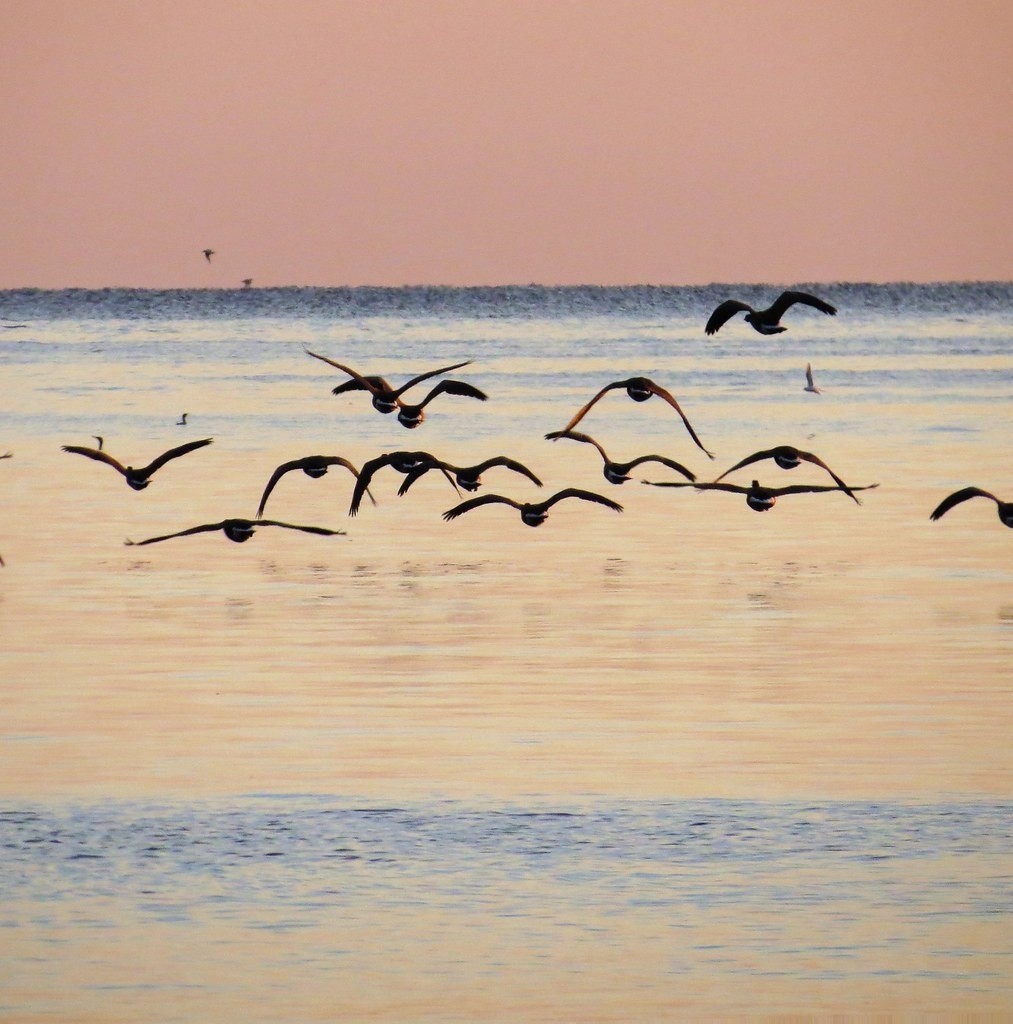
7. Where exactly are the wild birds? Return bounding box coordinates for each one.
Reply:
[397,455,543,498]
[442,488,625,527]
[543,431,697,485]
[803,363,825,395]
[304,346,474,414]
[714,445,862,506]
[331,376,488,429]
[256,456,378,520]
[57,438,215,491]
[347,451,463,518]
[929,485,1013,530]
[641,478,880,512]
[138,518,340,546]
[565,377,717,461]
[703,291,838,337]
[176,414,188,425]
[202,249,214,263]
